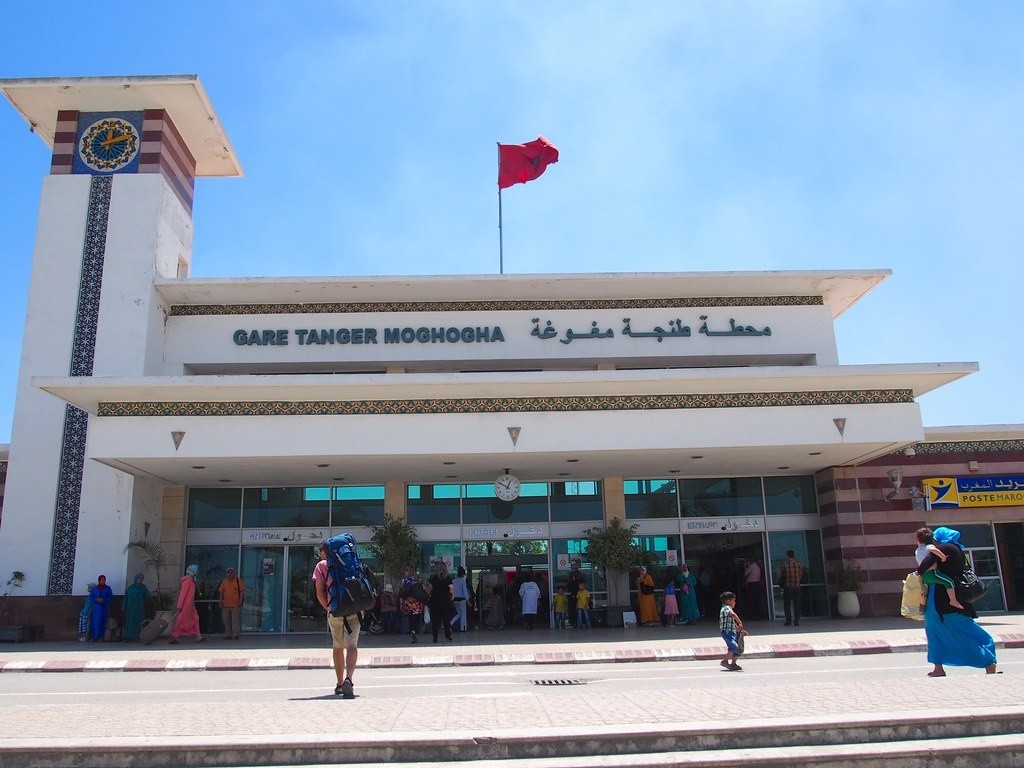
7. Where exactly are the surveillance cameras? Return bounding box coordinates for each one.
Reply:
[905,447,916,459]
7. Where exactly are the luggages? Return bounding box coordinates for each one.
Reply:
[139,609,182,644]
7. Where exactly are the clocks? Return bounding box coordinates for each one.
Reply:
[78,116,140,171]
[494,474,521,501]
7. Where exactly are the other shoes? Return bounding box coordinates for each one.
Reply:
[720,660,733,670]
[784,621,791,626]
[732,663,742,670]
[794,620,798,625]
[410,632,418,643]
[79,636,88,642]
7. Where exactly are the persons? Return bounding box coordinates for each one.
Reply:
[78,583,96,641]
[89,575,112,642]
[449,566,473,634]
[780,549,803,625]
[427,562,454,643]
[637,566,658,627]
[677,564,701,626]
[313,546,365,700]
[717,592,749,671]
[576,582,592,628]
[915,528,998,678]
[662,578,678,629]
[218,567,247,640]
[689,559,763,622]
[567,559,586,625]
[552,586,569,630]
[379,583,398,636]
[400,562,423,643]
[121,573,154,643]
[167,564,208,644]
[484,571,546,628]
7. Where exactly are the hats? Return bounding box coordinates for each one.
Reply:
[87,582,96,589]
[227,567,235,573]
[383,584,393,593]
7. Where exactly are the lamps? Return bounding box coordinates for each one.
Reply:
[884,467,903,502]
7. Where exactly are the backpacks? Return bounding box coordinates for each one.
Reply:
[323,533,377,617]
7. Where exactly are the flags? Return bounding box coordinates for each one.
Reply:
[498,135,559,189]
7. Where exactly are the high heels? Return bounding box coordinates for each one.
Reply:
[433,636,437,642]
[446,636,453,641]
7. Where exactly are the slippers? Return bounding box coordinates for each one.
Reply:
[196,637,207,643]
[167,638,179,645]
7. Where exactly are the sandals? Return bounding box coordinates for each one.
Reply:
[342,677,355,699]
[335,685,343,693]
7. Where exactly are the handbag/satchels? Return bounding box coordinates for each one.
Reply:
[368,621,384,634]
[424,605,430,625]
[400,582,412,599]
[901,573,928,620]
[640,576,653,594]
[412,584,428,601]
[953,570,988,604]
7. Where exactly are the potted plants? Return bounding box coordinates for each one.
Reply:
[0,571,25,643]
[580,514,660,628]
[832,564,865,618]
[119,538,174,637]
[370,516,423,632]
